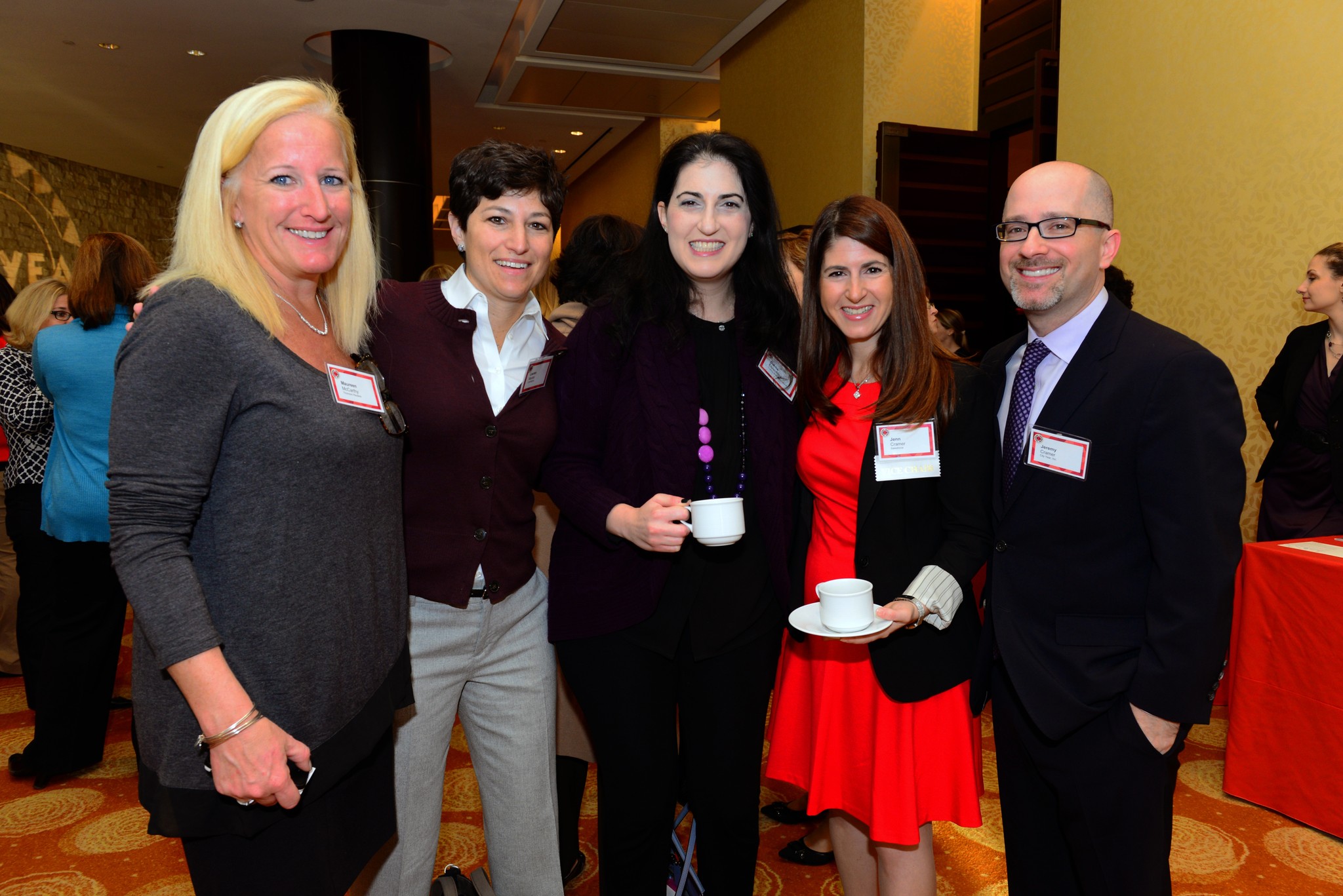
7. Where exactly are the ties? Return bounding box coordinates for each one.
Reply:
[994,339,1054,518]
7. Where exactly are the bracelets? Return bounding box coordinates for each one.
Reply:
[894,594,925,632]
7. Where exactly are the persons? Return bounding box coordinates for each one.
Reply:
[921,289,938,341]
[97,82,416,896]
[759,199,990,896]
[1249,241,1343,542]
[2,221,840,883]
[937,309,979,380]
[355,138,572,896]
[971,158,1247,895]
[544,123,809,896]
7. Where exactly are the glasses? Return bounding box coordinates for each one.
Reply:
[994,217,1114,244]
[47,308,75,322]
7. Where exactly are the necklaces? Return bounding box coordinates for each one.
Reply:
[1325,329,1343,360]
[844,360,881,401]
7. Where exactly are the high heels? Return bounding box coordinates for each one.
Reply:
[777,829,835,869]
[760,788,828,826]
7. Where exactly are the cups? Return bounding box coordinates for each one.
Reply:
[680,497,745,547]
[815,579,874,633]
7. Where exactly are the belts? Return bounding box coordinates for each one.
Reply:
[468,585,490,602]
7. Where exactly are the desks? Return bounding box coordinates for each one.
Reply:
[1219,536,1342,841]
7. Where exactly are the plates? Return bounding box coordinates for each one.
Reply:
[788,602,894,637]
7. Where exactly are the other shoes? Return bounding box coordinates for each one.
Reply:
[7,738,36,779]
[28,744,109,791]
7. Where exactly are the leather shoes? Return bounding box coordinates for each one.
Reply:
[555,848,586,889]
